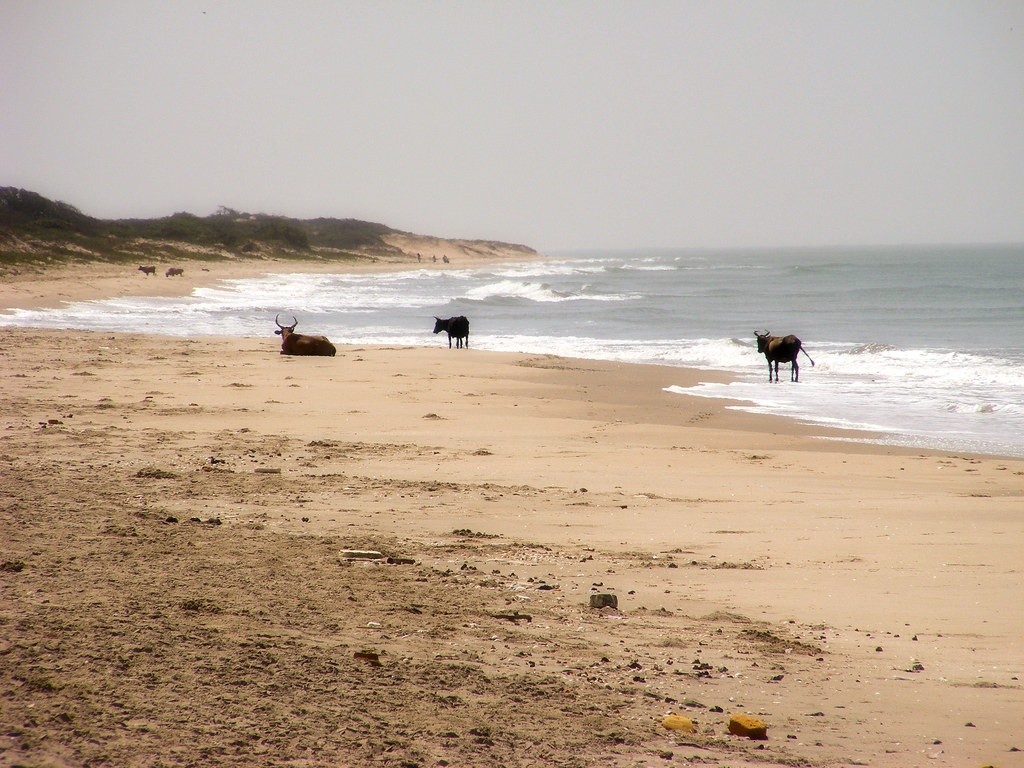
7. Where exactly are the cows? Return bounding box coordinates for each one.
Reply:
[754,329,815,382]
[274,313,337,357]
[433,316,469,349]
[138,265,184,277]
